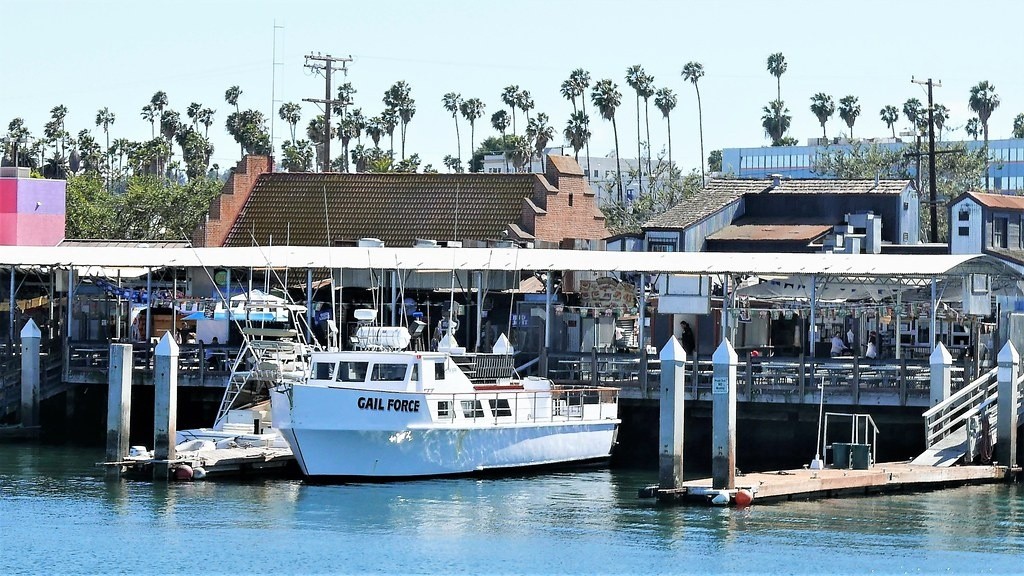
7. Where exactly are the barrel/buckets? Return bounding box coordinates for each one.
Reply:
[850,443,871,470]
[832,444,849,469]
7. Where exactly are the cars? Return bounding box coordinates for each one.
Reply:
[139,307,197,339]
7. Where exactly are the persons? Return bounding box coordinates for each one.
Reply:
[205,337,222,371]
[677,321,695,355]
[865,331,883,359]
[176,322,195,344]
[831,331,848,357]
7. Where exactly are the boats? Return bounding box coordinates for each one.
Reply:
[127,230,356,479]
[269,275,622,485]
